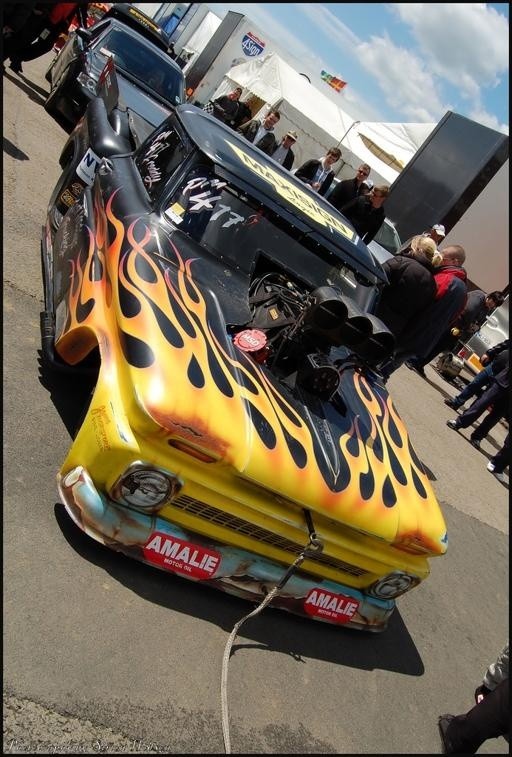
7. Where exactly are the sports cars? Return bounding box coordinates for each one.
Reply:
[38,96,454,637]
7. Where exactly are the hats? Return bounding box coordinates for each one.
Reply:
[432,223,446,239]
[285,131,298,143]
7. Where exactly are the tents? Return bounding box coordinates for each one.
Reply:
[203,50,403,212]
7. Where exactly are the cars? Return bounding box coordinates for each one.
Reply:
[295,163,402,266]
[43,2,187,131]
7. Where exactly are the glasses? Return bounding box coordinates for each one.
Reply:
[358,169,368,177]
[371,190,384,198]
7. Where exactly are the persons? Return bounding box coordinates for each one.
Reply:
[475,642,509,706]
[1,0,88,78]
[437,675,512,756]
[214,88,509,477]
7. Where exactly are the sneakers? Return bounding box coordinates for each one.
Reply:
[493,470,506,484]
[446,419,460,431]
[443,398,459,412]
[414,366,427,378]
[439,713,455,753]
[487,459,496,473]
[471,437,481,449]
[404,359,414,370]
